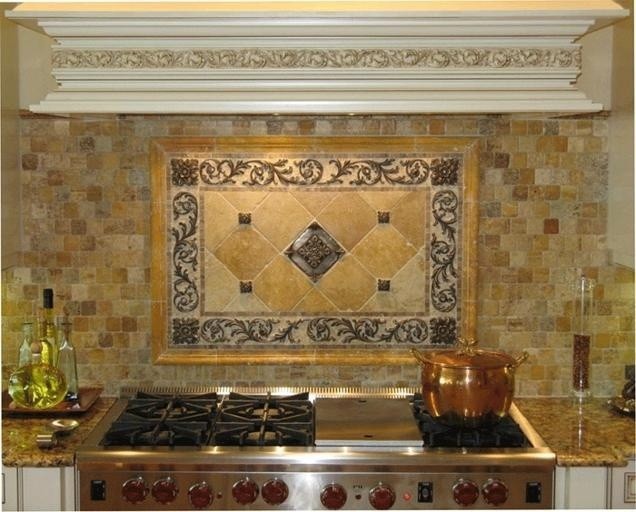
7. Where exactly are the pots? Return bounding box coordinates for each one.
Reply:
[405,336,530,420]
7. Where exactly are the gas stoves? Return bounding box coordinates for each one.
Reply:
[76,386,557,511]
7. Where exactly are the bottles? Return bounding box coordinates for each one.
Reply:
[567,275,596,404]
[7,286,78,410]
